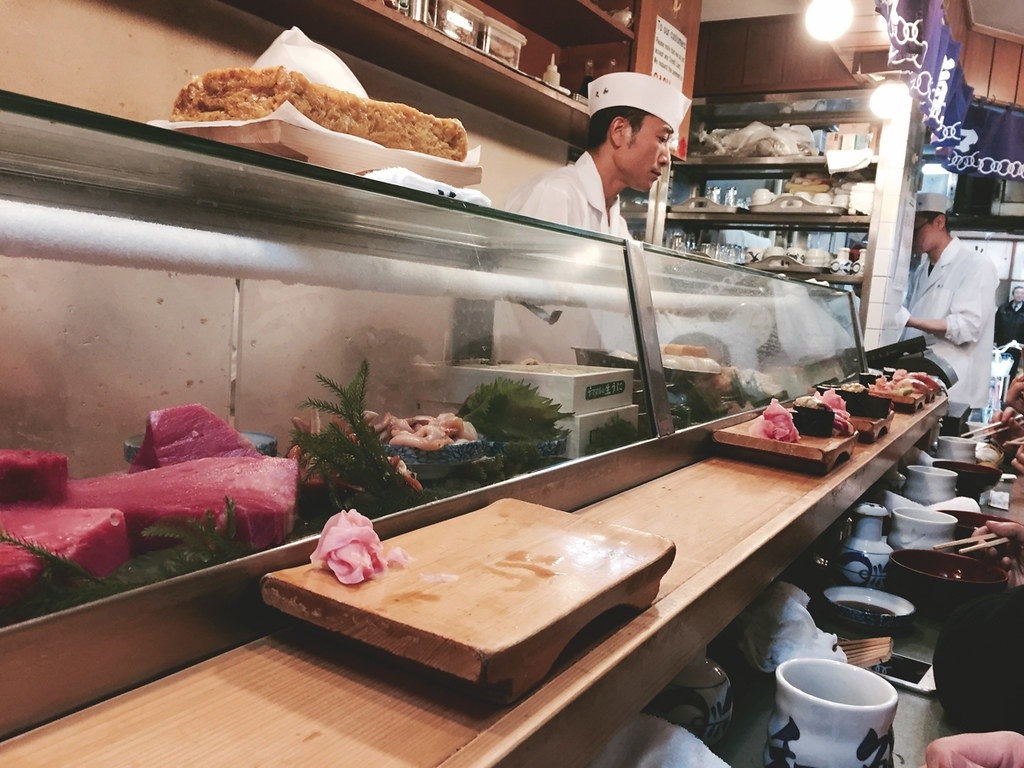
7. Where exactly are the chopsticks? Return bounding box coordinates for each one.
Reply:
[960,414,1024,441]
[933,533,1012,555]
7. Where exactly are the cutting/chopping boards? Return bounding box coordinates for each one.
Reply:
[261,497,678,701]
[171,123,484,187]
[711,369,945,476]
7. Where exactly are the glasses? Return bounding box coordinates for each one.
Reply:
[914,219,930,237]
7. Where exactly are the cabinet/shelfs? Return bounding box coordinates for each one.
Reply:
[652,102,885,341]
[218,0,697,156]
[1,87,952,767]
[620,180,657,243]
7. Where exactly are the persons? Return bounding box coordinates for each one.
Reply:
[491,72,695,366]
[994,286,1024,346]
[925,371,1024,768]
[901,171,998,410]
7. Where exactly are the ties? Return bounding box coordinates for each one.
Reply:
[1013,304,1017,314]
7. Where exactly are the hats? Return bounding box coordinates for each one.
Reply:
[912,163,958,214]
[587,72,692,136]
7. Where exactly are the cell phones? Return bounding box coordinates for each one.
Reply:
[871,651,937,695]
[1000,473,1018,484]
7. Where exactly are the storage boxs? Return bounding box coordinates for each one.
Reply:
[436,0,482,50]
[478,17,527,69]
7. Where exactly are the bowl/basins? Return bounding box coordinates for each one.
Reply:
[901,464,959,509]
[823,583,918,634]
[888,547,1007,622]
[932,460,1003,503]
[762,661,896,768]
[886,508,960,555]
[935,435,978,465]
